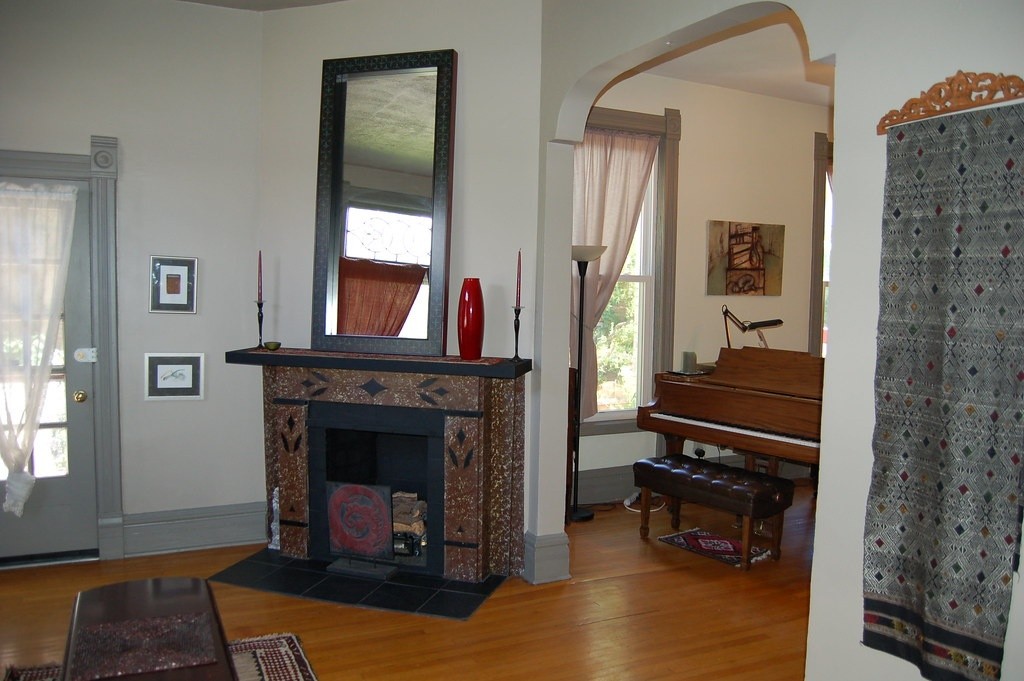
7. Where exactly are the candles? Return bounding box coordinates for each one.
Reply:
[255,248,266,302]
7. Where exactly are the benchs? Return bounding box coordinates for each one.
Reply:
[631,449,795,567]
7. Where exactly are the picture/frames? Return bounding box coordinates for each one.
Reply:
[142,352,206,401]
[704,220,787,298]
[146,252,200,316]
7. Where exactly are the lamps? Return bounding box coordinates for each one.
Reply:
[568,243,609,526]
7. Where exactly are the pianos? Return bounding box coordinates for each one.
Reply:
[637,345,826,513]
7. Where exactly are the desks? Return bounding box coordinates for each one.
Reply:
[61,575,244,681]
[223,339,532,588]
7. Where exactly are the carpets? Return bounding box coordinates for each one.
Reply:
[2,632,320,681]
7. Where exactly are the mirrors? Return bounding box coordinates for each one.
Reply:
[310,47,460,355]
